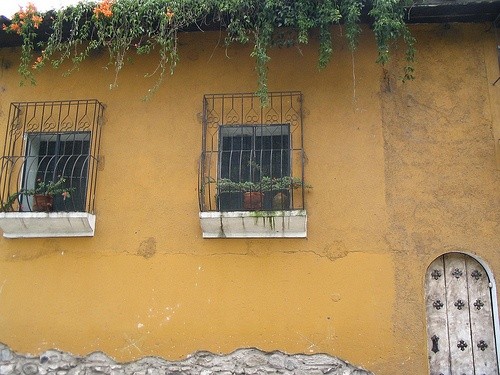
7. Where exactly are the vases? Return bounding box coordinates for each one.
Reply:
[31,195,53,212]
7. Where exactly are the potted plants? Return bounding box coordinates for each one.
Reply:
[263,175,313,209]
[199,174,269,210]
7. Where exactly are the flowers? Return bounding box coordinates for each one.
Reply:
[2,175,78,209]
